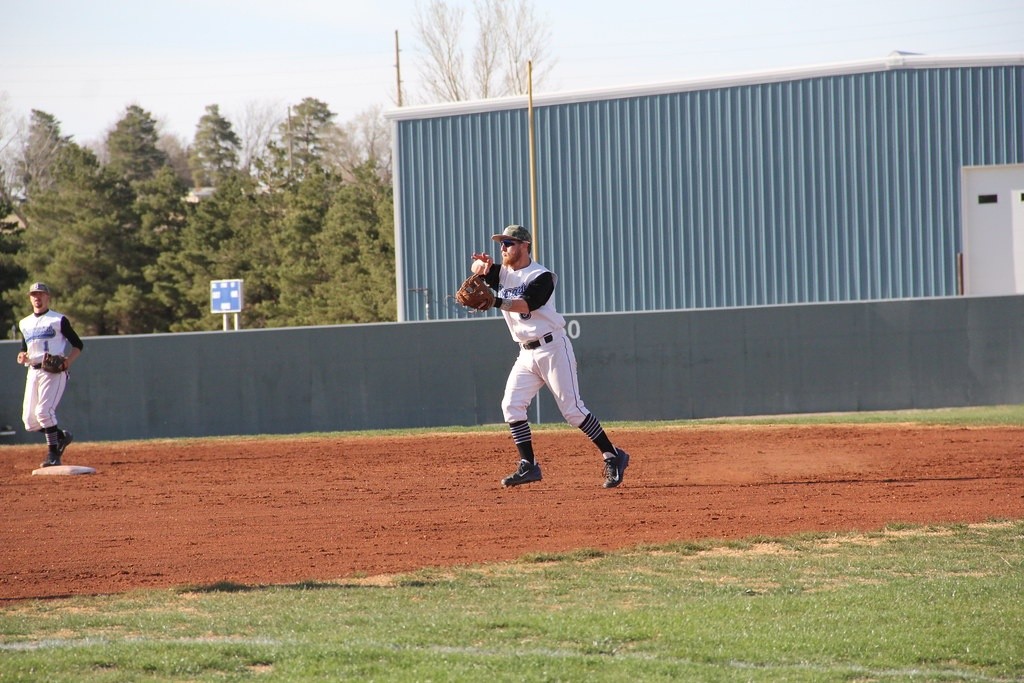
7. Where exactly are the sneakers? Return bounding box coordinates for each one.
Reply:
[501,459,541,488]
[602,448,629,488]
[57,430,72,456]
[41,451,60,467]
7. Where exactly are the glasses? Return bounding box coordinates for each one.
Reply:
[500,239,530,247]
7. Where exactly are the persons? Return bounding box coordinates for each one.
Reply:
[455,224,629,488]
[17,282,84,468]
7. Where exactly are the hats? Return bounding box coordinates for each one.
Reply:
[492,225,532,244]
[29,283,48,292]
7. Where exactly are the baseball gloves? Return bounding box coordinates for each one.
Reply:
[454,273,495,311]
[41,352,68,374]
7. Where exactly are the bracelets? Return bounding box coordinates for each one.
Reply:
[500,298,512,311]
[493,297,502,308]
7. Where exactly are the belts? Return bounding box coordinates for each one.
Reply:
[33,364,41,369]
[523,335,552,349]
